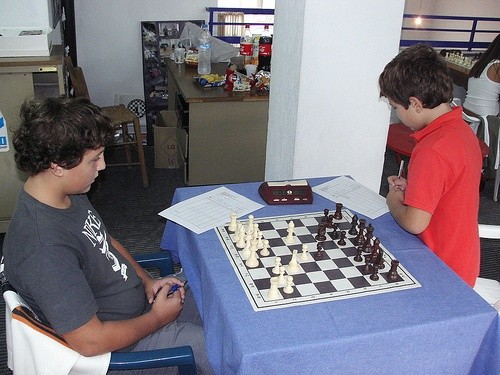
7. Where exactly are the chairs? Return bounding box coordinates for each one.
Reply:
[65,56,149,190]
[449,97,500,202]
[472,224,500,314]
[0,249,198,375]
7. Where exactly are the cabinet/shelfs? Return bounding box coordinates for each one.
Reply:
[0,41,69,232]
[164,56,269,187]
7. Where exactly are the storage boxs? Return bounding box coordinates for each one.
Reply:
[51,16,64,47]
[0,27,53,58]
[152,109,179,170]
[0,0,62,30]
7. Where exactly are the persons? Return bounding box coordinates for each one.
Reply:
[462,34,500,183]
[378,42,483,289]
[3,94,213,375]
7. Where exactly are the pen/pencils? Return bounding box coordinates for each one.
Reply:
[397,159,404,178]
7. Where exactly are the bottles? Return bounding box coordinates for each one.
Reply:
[198,25,211,75]
[258,25,272,72]
[240,24,253,64]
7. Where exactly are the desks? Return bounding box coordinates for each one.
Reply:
[387,123,490,177]
[158,175,500,375]
[438,50,485,88]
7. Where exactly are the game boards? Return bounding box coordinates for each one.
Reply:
[213,209,422,312]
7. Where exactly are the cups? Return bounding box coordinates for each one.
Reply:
[231,56,243,70]
[174,48,185,63]
[245,65,257,76]
